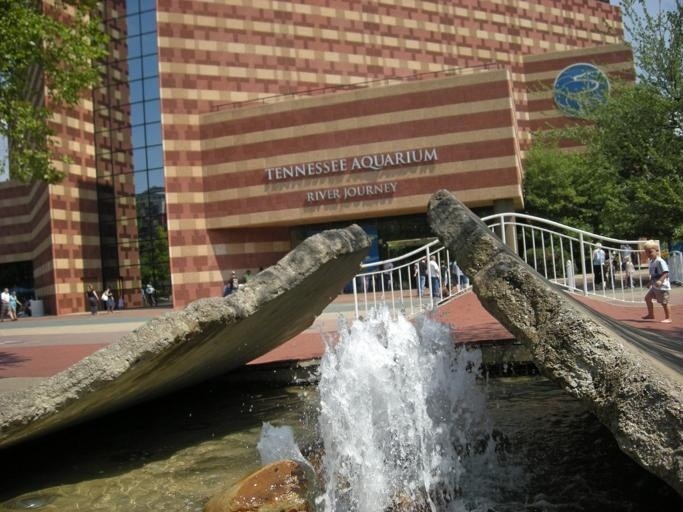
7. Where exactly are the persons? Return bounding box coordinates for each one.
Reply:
[146,280,158,307]
[383,263,393,290]
[86,283,126,315]
[223,266,266,296]
[619,243,635,289]
[0,288,14,321]
[566,256,575,292]
[639,245,673,323]
[10,291,22,320]
[592,242,607,291]
[413,253,470,298]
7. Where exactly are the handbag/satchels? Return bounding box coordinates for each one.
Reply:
[101,293,108,301]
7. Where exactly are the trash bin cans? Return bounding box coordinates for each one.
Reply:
[27,299,43,317]
